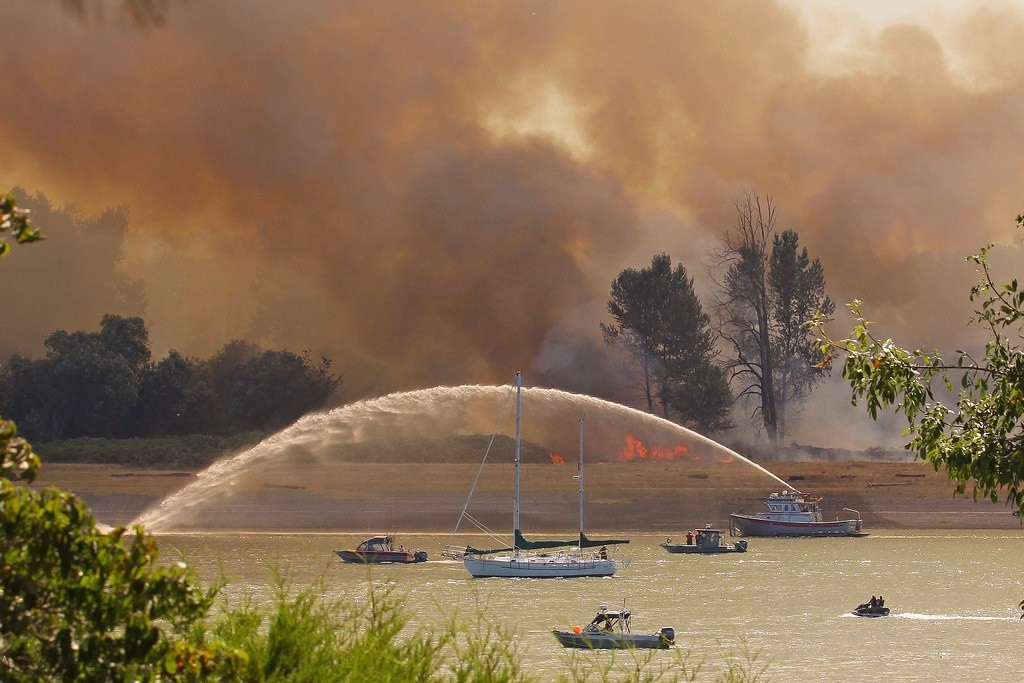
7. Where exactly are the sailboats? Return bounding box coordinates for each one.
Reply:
[440,367,631,580]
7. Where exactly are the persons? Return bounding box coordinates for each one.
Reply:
[399,545,405,553]
[598,545,610,560]
[685,531,703,545]
[868,595,885,610]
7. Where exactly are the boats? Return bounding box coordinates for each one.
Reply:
[332,534,430,565]
[726,488,871,538]
[852,603,892,619]
[657,522,749,556]
[550,604,677,651]
